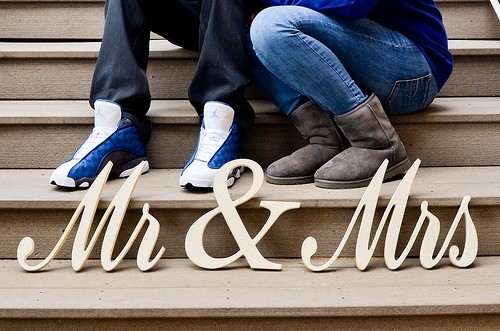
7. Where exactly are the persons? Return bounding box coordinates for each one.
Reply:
[51,1,268,189]
[245,0,453,190]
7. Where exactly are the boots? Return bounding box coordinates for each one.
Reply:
[265,92,412,189]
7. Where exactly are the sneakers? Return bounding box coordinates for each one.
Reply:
[49,98,149,188]
[180,101,244,191]
